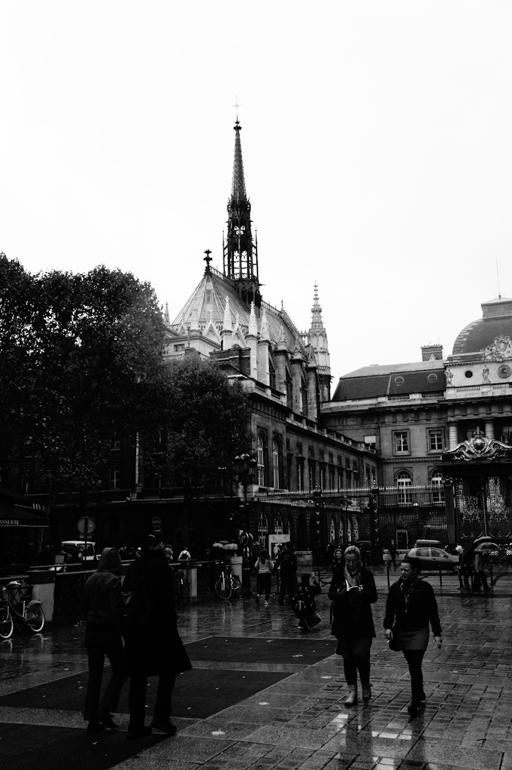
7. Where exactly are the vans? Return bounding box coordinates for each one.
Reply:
[61,540,96,560]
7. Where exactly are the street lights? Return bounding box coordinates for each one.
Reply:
[234,453,258,598]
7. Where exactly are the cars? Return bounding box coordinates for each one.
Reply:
[405,547,461,574]
[474,536,512,561]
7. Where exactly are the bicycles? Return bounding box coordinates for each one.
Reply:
[0,581,44,638]
[215,565,233,600]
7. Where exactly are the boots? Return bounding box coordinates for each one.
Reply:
[152,700,179,734]
[361,678,372,700]
[344,685,357,704]
[128,709,153,739]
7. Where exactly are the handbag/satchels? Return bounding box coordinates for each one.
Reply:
[388,622,403,652]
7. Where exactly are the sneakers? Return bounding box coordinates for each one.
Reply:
[100,713,121,729]
[87,721,105,733]
[407,697,426,716]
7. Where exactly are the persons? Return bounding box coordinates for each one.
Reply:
[272,546,296,606]
[285,541,298,571]
[122,532,196,740]
[386,543,399,569]
[328,545,379,706]
[254,550,276,606]
[382,556,442,714]
[177,543,193,579]
[288,572,322,632]
[81,546,127,733]
[473,550,488,583]
[331,548,345,579]
[457,543,474,591]
